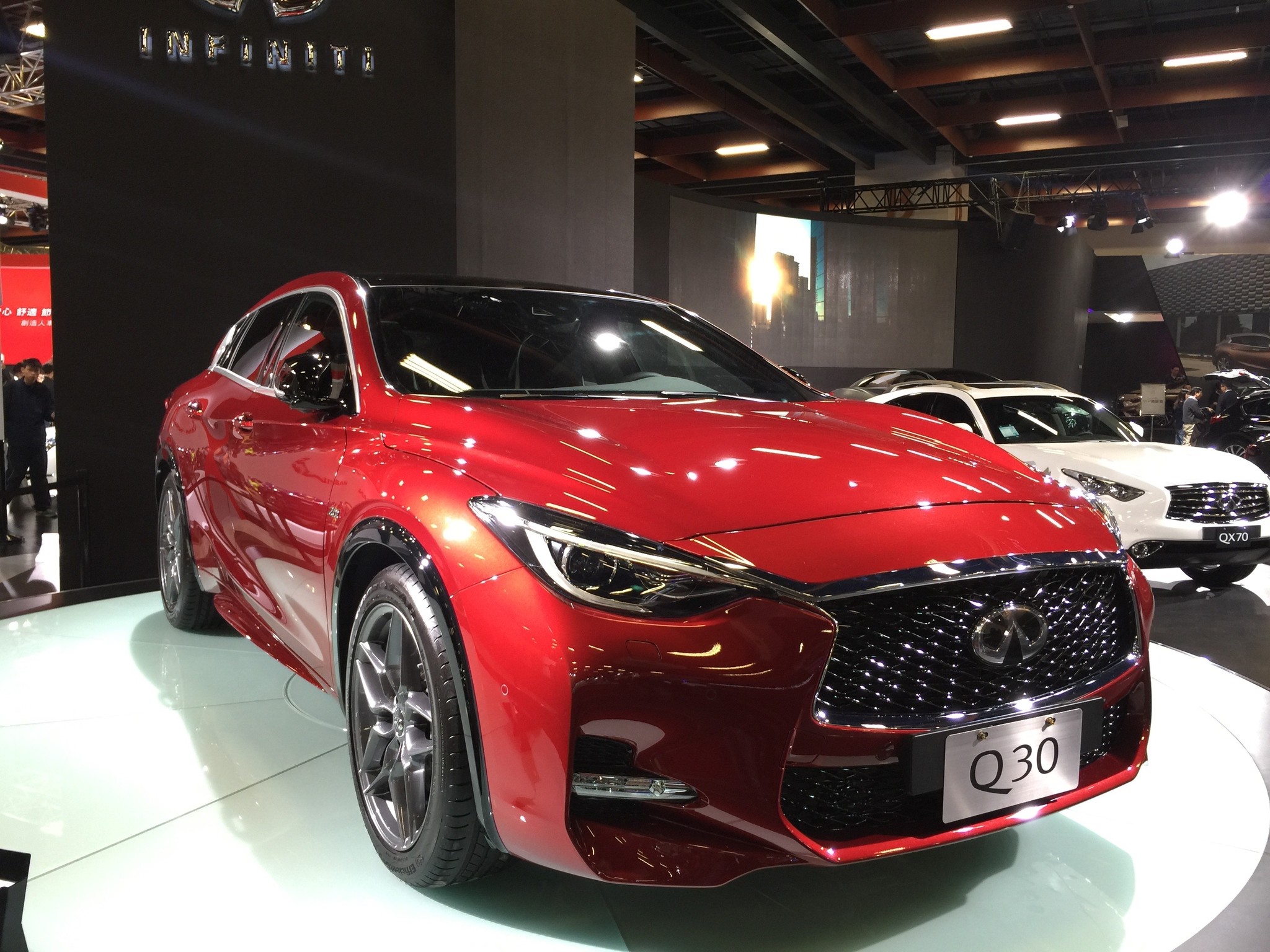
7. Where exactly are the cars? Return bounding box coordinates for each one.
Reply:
[1201,368,1270,400]
[829,367,1004,403]
[1110,377,1192,429]
[155,269,1158,898]
[25,423,57,498]
[1212,332,1270,379]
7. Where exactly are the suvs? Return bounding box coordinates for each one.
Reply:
[1203,389,1270,477]
[862,378,1270,588]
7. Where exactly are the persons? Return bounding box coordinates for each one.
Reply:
[1173,389,1191,446]
[1181,386,1216,446]
[4,362,26,387]
[1164,364,1185,390]
[40,363,56,428]
[2,357,59,518]
[1182,384,1193,391]
[1213,379,1238,415]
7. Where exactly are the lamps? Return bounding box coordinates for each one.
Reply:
[1056,197,1077,233]
[1086,193,1109,231]
[1124,192,1155,234]
[1162,49,1249,68]
[715,142,770,158]
[634,70,644,84]
[995,111,1062,127]
[924,14,1014,42]
